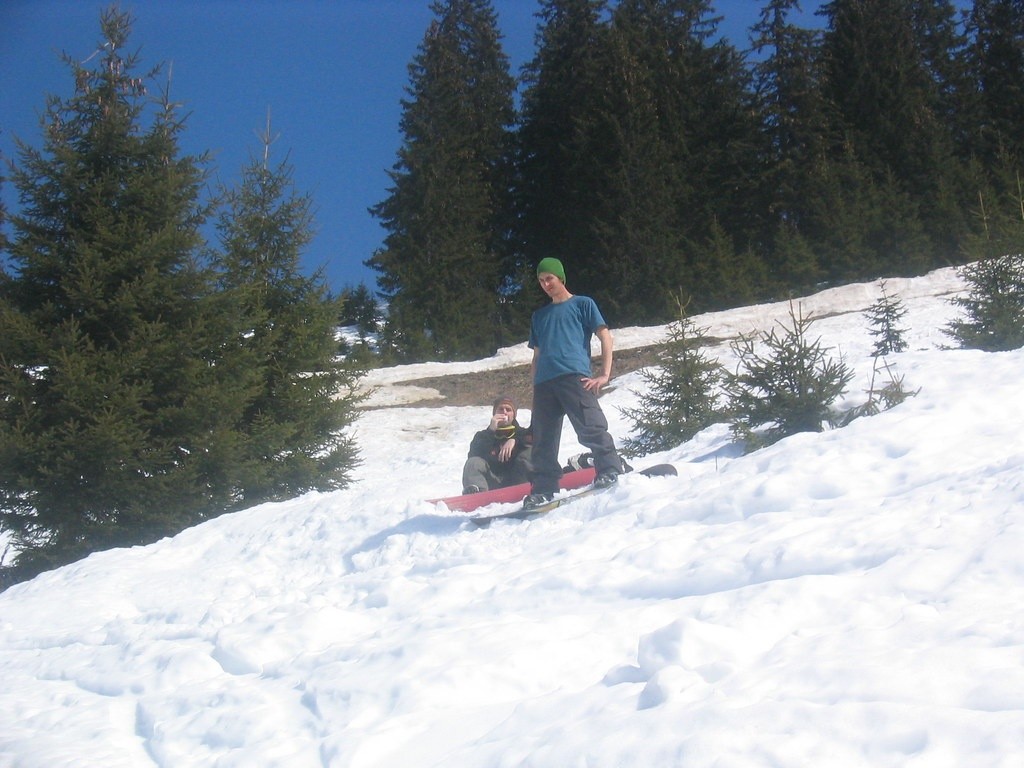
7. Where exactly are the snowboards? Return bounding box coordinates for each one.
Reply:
[468,477,621,527]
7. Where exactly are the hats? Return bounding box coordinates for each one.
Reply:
[493,396,517,418]
[537,257,566,284]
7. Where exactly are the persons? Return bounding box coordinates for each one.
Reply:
[463,396,535,494]
[523,257,614,511]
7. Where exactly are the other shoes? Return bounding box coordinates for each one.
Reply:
[462,485,479,495]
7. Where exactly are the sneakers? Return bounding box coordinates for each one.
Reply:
[523,493,552,508]
[594,472,619,489]
